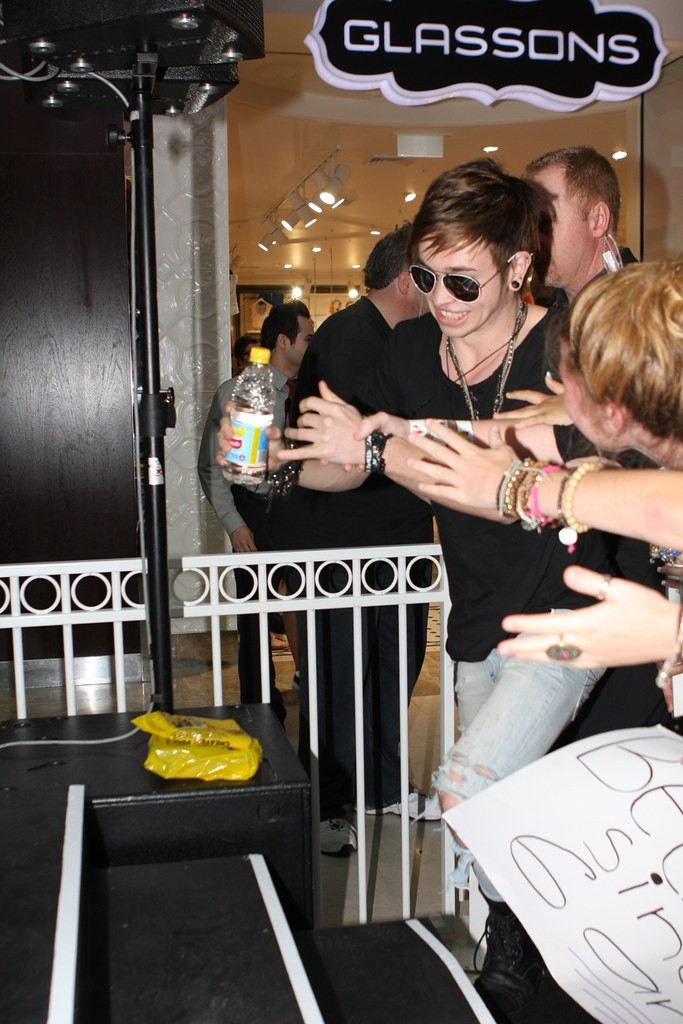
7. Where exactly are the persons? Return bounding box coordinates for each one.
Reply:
[284,225,444,859]
[214,157,648,1024]
[235,335,259,377]
[198,300,317,699]
[524,145,646,744]
[321,258,683,1023]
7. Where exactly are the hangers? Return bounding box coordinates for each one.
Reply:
[249,294,274,308]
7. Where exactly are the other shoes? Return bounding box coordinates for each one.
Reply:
[293,671,301,690]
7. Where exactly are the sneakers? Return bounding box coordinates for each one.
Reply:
[270,632,289,649]
[355,782,442,819]
[320,816,358,855]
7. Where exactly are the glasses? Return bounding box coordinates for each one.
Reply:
[408,251,522,303]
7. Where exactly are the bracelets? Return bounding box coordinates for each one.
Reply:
[274,436,303,498]
[498,459,561,527]
[409,418,427,443]
[456,420,473,444]
[555,461,607,537]
[432,417,448,445]
[363,429,389,475]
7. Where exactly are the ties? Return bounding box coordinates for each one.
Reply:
[285,378,297,427]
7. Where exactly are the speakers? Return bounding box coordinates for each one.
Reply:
[0,0,265,115]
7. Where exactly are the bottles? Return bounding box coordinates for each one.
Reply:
[221,346,276,483]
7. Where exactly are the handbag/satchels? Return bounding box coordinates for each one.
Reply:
[129,712,263,780]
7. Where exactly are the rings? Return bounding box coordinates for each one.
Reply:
[217,450,225,457]
[597,574,611,600]
[544,632,582,660]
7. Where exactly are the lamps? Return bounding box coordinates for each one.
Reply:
[257,145,347,251]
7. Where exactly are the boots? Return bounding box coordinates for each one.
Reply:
[474,885,544,1014]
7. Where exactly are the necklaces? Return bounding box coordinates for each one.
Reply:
[442,298,528,427]
